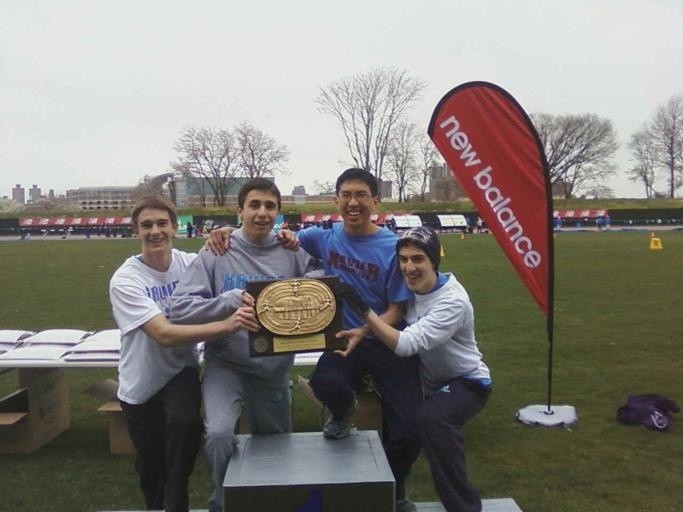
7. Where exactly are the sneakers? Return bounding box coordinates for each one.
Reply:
[323,391,357,440]
[396,498,416,512]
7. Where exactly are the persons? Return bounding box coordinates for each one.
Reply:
[595,214,611,234]
[17,216,493,235]
[556,215,563,229]
[108,194,300,512]
[203,166,424,511]
[166,176,323,512]
[331,226,493,512]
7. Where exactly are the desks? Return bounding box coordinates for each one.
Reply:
[1,351,322,366]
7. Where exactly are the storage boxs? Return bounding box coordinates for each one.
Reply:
[81,376,139,455]
[0,367,70,455]
[296,373,384,432]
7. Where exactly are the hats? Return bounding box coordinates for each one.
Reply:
[398,227,442,265]
